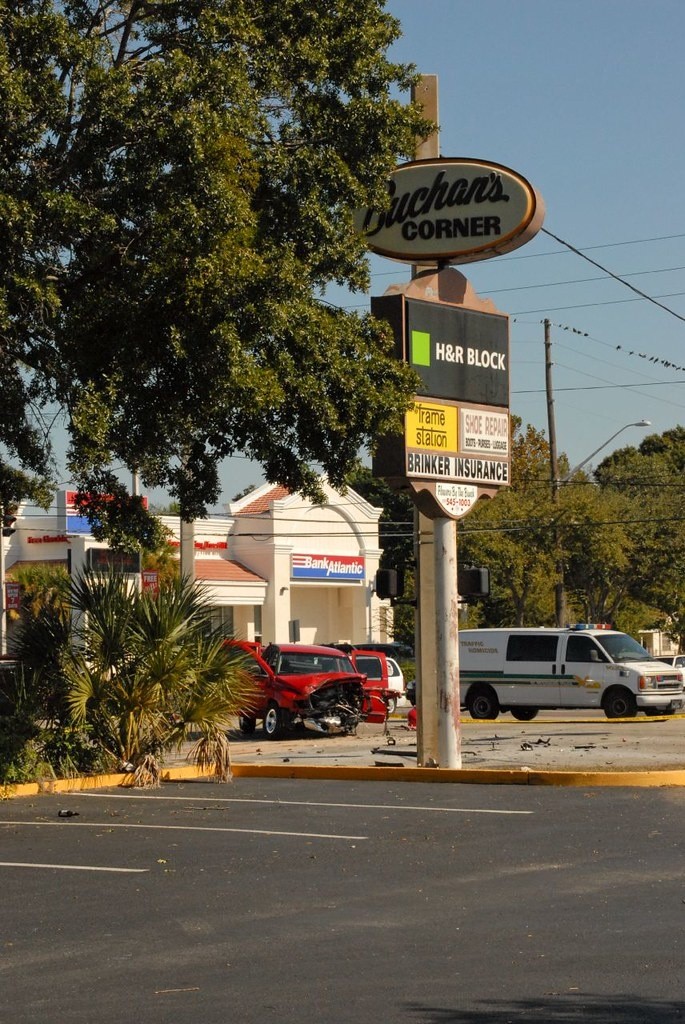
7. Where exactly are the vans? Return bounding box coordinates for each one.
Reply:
[458,624,685,722]
[314,654,404,715]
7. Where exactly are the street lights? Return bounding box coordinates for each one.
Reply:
[546,420,651,628]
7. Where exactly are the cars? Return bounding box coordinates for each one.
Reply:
[653,655,685,688]
[218,639,402,739]
[405,680,415,707]
